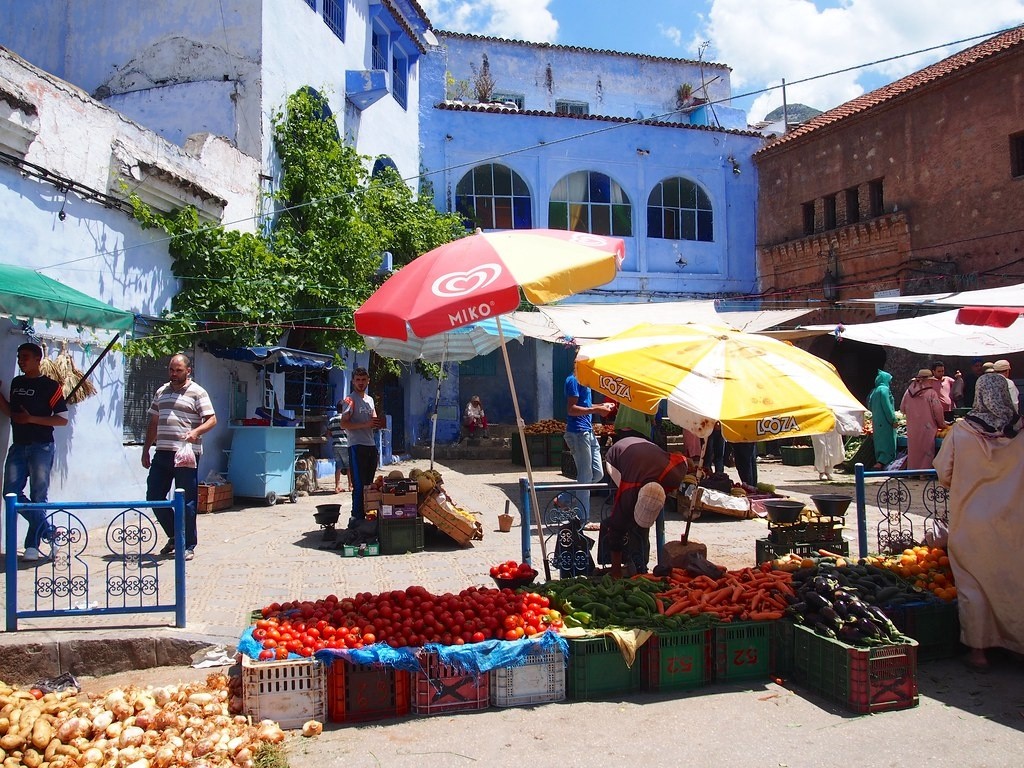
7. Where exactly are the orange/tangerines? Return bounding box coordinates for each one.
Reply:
[890,544,959,600]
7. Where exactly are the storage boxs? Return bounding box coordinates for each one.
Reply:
[512,432,875,478]
[241,478,965,731]
[953,407,973,421]
[197,483,232,514]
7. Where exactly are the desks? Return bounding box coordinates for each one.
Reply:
[896,435,944,480]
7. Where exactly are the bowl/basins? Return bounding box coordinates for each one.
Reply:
[489,570,539,591]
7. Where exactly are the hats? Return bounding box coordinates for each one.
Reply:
[982,362,994,373]
[911,368,938,382]
[634,483,665,529]
[994,360,1012,371]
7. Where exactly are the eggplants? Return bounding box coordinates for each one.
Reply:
[769,565,906,647]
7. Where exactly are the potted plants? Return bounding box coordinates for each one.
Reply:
[472,73,496,103]
[676,83,694,105]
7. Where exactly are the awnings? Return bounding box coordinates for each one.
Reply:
[0,264,135,404]
[197,338,335,426]
[502,283,1024,356]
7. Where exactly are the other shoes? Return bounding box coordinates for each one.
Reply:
[160,539,176,554]
[483,435,490,439]
[347,518,358,529]
[185,548,193,560]
[826,472,835,481]
[819,473,823,481]
[584,521,599,530]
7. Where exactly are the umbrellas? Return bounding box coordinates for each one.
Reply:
[351,225,627,580]
[574,323,867,546]
[362,314,525,472]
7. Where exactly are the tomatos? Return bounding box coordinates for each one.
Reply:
[252,561,562,662]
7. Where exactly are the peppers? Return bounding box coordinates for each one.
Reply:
[516,573,720,631]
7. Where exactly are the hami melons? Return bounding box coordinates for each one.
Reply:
[388,468,433,493]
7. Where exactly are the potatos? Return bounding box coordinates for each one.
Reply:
[523,419,568,434]
[0,680,75,768]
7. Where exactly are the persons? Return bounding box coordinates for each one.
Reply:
[465,396,489,438]
[326,399,354,493]
[983,359,1019,415]
[604,437,688,580]
[932,372,1024,672]
[682,424,731,474]
[811,427,846,481]
[603,393,662,442]
[869,369,899,471]
[0,343,69,561]
[564,356,611,530]
[340,365,382,527]
[142,355,218,561]
[930,360,962,418]
[899,369,948,480]
[734,443,757,486]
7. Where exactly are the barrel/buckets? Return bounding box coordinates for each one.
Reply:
[498,514,514,532]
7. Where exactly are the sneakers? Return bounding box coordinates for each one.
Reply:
[23,546,37,561]
[49,529,65,558]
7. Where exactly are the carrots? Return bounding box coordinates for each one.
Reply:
[631,549,846,624]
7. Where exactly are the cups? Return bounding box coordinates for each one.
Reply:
[230,420,244,426]
[372,417,378,429]
[243,418,269,426]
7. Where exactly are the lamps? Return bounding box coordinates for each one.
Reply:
[676,253,687,266]
[447,133,453,141]
[822,264,837,305]
[728,154,741,175]
[637,147,649,155]
[539,141,546,145]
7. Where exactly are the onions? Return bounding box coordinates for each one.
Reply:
[56,671,322,768]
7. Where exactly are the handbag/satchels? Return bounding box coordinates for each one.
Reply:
[597,513,643,564]
[174,434,198,469]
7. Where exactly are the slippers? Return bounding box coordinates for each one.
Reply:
[964,649,989,674]
